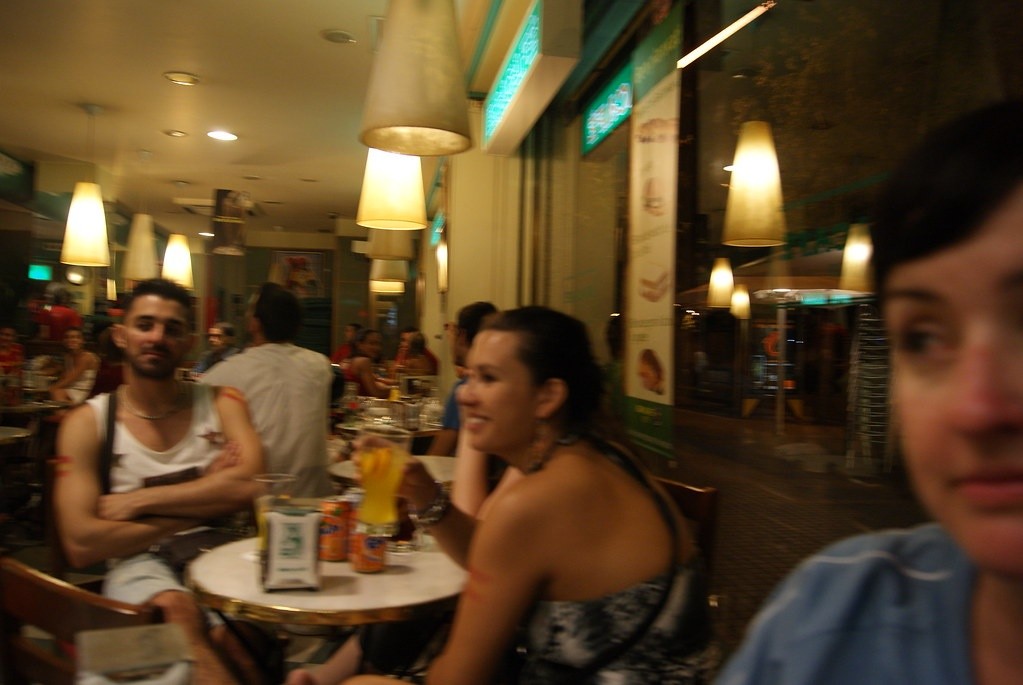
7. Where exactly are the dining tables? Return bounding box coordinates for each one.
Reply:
[328,455,456,495]
[335,419,441,456]
[0,396,56,537]
[183,535,470,684]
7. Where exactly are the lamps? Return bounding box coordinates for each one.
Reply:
[703,67,875,322]
[59,104,196,290]
[354,0,472,296]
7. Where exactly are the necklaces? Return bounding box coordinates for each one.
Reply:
[119,380,182,421]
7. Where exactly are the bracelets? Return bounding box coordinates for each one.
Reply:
[408,485,452,531]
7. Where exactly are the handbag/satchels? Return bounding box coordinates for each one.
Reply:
[159,529,231,571]
[189,606,280,685]
[492,652,595,685]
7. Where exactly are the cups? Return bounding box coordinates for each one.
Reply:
[250,471,301,579]
[352,423,414,538]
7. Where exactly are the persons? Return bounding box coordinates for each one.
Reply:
[722,94,1022,684]
[291,295,720,684]
[1,280,440,427]
[55,282,268,684]
[194,282,332,497]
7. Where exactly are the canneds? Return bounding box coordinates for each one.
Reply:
[319,496,387,574]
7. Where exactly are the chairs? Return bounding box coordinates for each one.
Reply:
[1,556,163,685]
